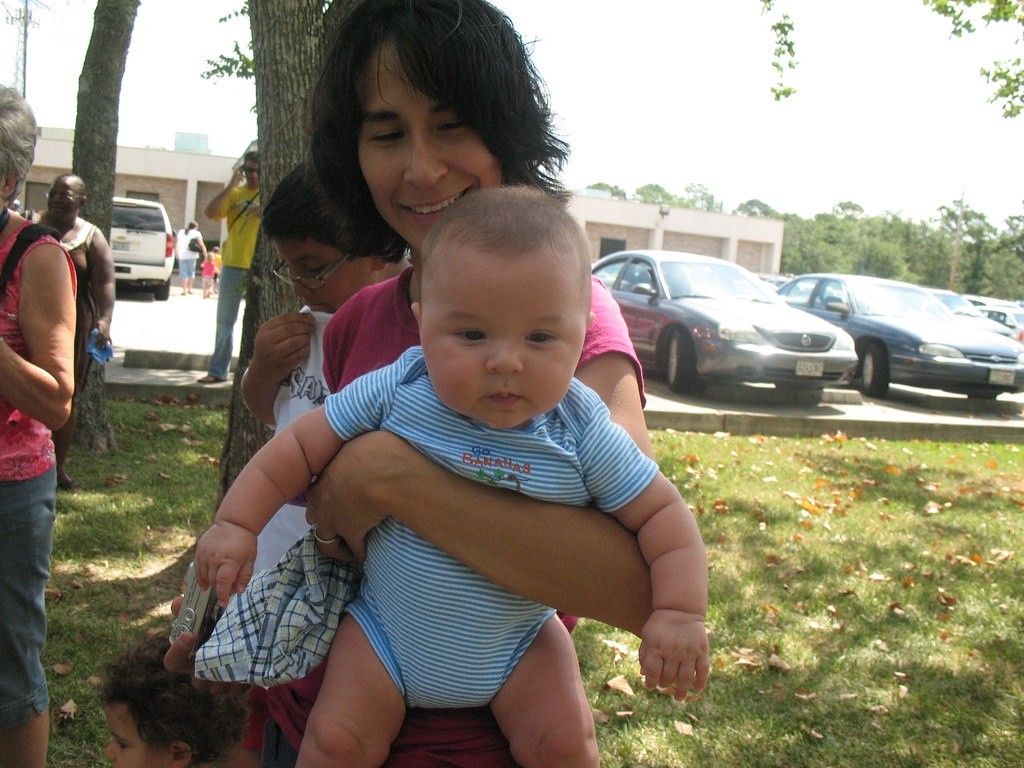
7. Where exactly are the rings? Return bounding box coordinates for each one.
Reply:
[313,527,337,545]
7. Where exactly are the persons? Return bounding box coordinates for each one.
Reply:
[102,0,709,767]
[174,220,208,295]
[209,246,222,293]
[200,254,219,298]
[198,150,263,384]
[0,84,115,768]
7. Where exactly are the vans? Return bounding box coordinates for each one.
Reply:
[110,196,176,300]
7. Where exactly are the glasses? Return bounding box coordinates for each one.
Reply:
[46,192,74,201]
[241,165,259,172]
[273,252,353,290]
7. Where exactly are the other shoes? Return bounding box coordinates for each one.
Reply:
[57,479,80,491]
[199,375,224,382]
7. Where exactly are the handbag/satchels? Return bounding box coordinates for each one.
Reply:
[188,239,202,251]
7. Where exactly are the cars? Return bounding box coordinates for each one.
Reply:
[777,272,1024,401]
[591,250,859,407]
